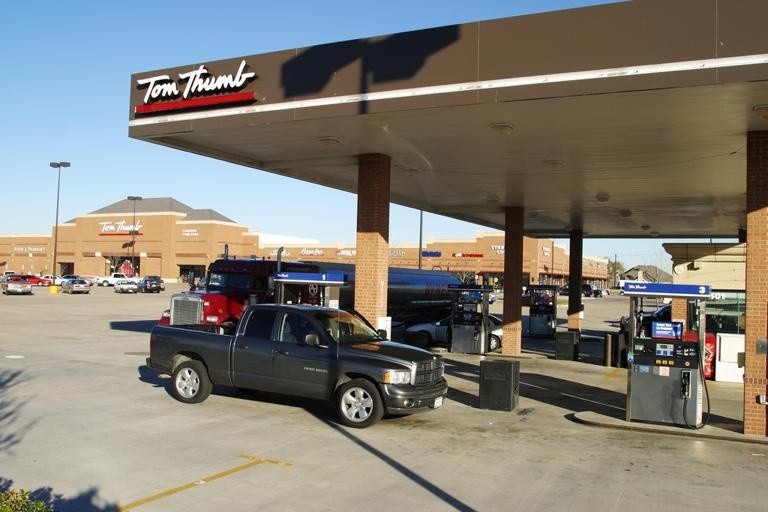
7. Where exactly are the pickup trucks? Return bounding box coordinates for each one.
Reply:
[142,302,449,430]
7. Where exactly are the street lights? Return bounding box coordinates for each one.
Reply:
[125,195,143,278]
[49,160,72,286]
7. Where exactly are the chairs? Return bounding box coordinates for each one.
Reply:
[282,322,296,342]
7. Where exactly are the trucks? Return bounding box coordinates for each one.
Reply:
[156,242,468,330]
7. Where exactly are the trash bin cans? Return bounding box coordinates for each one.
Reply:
[479,359,520,412]
[556,331,579,361]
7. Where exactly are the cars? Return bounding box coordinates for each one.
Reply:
[401,309,504,352]
[195,276,219,290]
[0,269,166,297]
[460,281,603,308]
[618,302,671,337]
